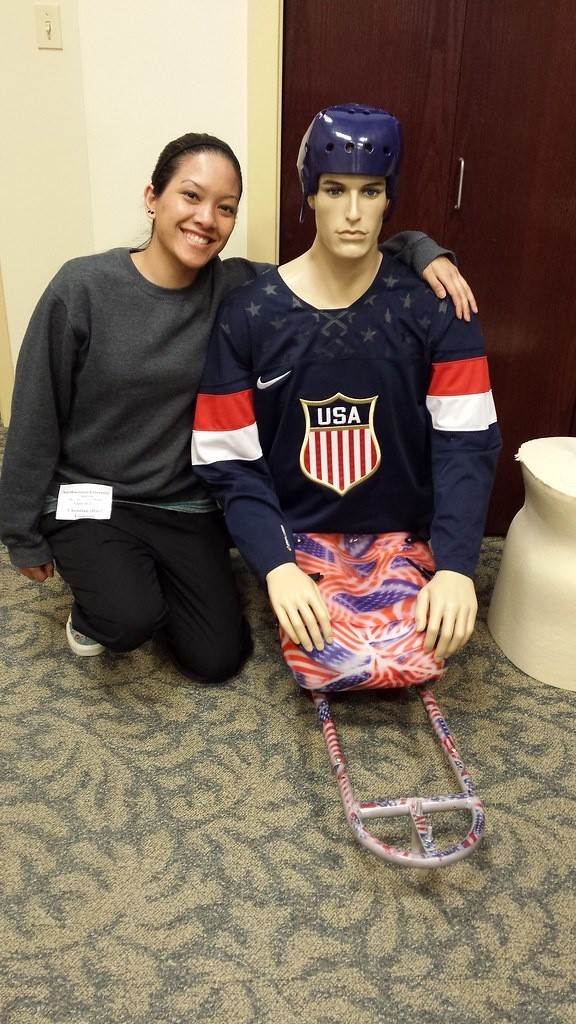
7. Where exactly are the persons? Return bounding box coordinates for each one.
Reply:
[1,131,277,687]
[187,101,507,694]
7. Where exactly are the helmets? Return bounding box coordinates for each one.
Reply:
[298,103,403,225]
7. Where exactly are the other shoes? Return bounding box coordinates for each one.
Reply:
[65,609,106,659]
